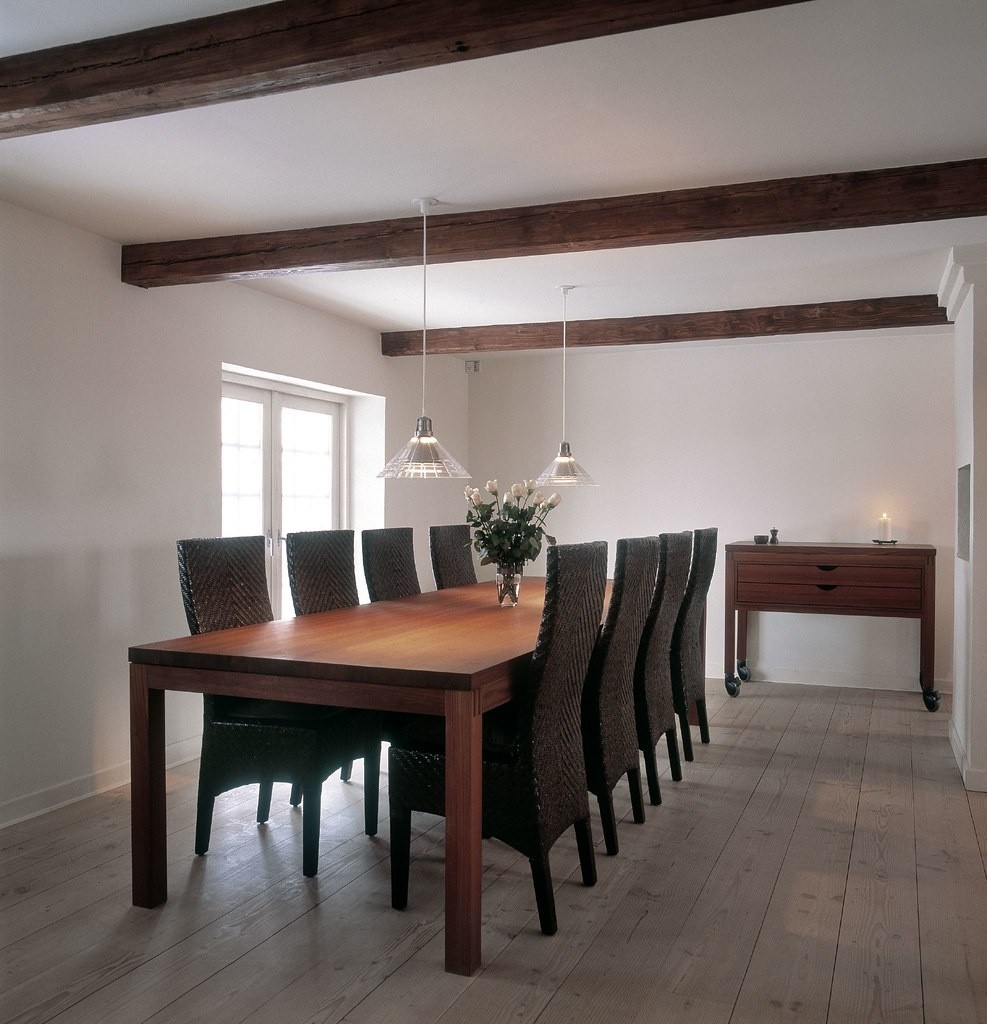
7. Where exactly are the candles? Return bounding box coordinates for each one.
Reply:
[879,513,892,541]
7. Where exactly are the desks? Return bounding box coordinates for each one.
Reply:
[127,575,616,978]
[723,541,943,713]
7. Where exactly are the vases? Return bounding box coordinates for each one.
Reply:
[495,563,522,609]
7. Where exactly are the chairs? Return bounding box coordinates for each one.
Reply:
[389,539,609,937]
[361,527,422,602]
[178,536,381,878]
[286,529,362,808]
[669,527,719,761]
[632,529,693,806]
[580,535,661,856]
[429,525,479,591]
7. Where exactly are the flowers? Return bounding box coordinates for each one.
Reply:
[462,479,562,566]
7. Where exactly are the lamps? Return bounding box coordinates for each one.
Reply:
[533,276,601,488]
[374,195,476,480]
